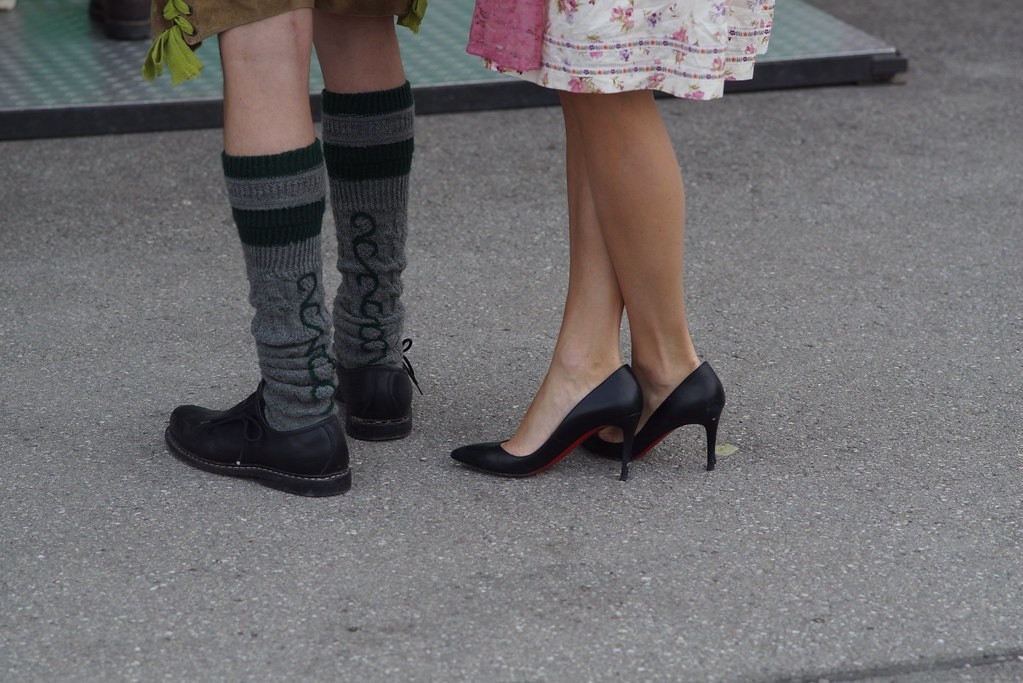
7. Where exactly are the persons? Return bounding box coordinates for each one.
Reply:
[449,0,774,483]
[141,0,430,497]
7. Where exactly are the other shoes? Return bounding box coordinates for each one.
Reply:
[88,0,151,40]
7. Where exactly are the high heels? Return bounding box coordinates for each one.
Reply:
[581,361,725,471]
[449,363,644,482]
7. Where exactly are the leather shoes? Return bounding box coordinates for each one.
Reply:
[164,378,352,497]
[336,338,422,441]
